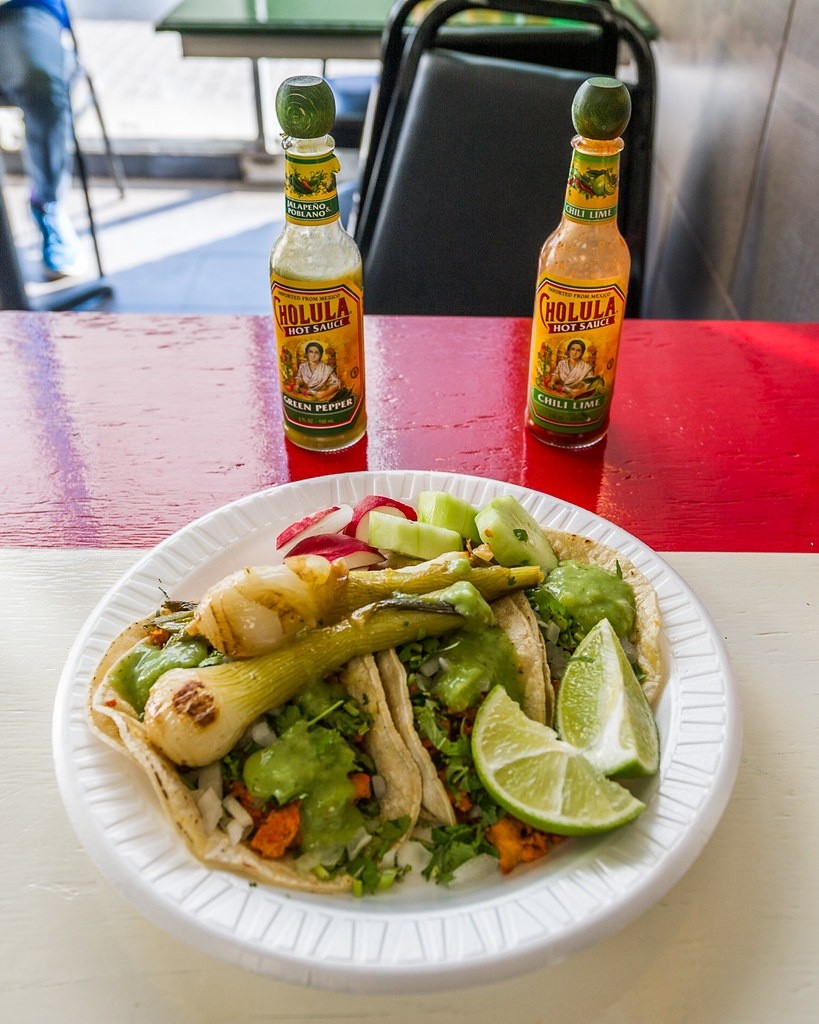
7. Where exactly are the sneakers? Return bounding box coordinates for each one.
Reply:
[31,207,79,278]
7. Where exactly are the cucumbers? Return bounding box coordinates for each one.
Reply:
[361,488,561,574]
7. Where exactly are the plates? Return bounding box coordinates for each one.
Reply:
[50,470,738,991]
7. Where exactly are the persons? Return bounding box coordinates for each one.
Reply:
[0,0,73,281]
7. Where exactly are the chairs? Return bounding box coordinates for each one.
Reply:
[348,0,660,317]
[0,22,126,278]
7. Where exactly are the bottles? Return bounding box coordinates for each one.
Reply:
[524,76,630,445]
[269,76,369,451]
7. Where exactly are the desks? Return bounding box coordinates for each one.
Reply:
[0,311,818,1024]
[157,0,657,150]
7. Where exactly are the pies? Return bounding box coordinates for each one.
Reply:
[87,527,664,891]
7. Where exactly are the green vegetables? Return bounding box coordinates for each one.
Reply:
[212,628,507,891]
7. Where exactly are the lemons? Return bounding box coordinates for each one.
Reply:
[469,683,645,838]
[558,616,661,778]
